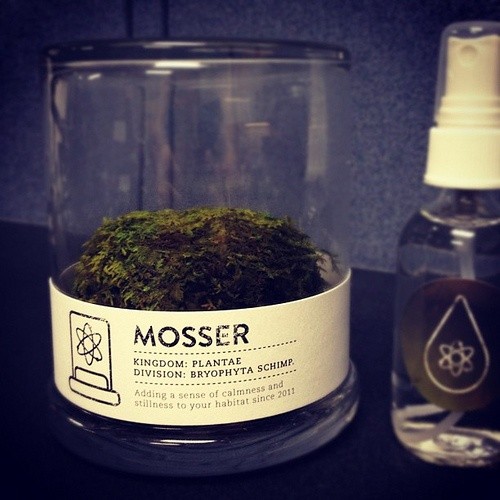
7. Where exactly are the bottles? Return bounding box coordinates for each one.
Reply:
[391,23,500,466]
